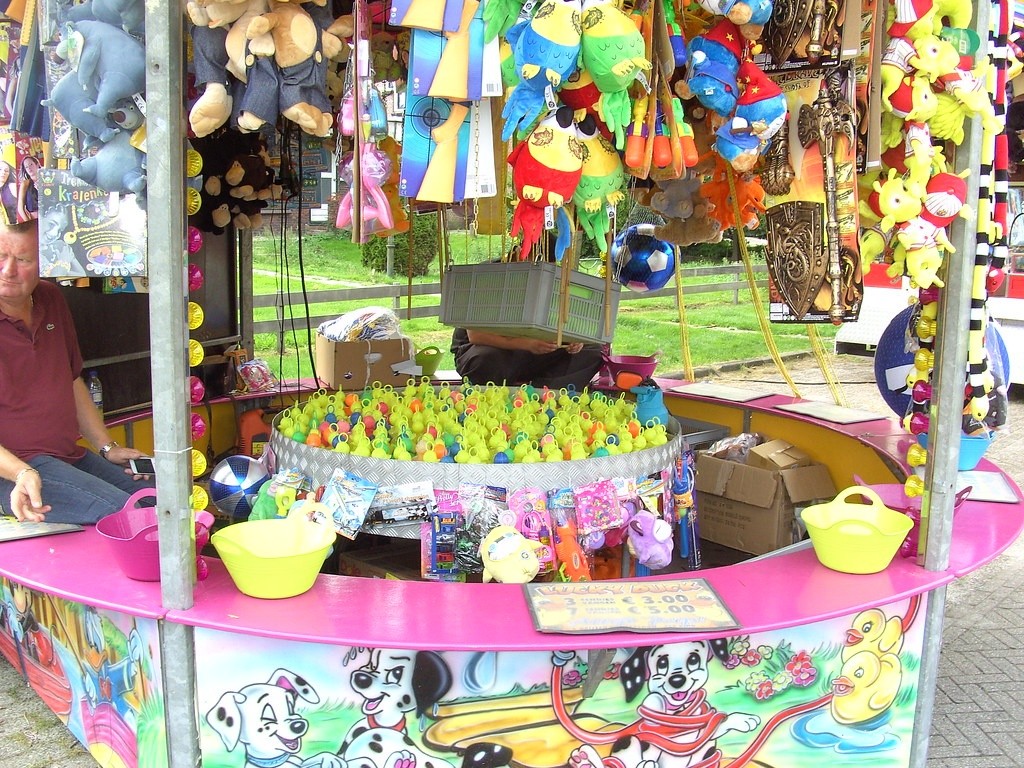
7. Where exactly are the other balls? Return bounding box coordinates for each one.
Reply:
[873,302,1012,420]
[210,455,271,518]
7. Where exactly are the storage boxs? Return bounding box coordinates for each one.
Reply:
[665,414,729,450]
[192,481,236,546]
[745,438,811,472]
[339,541,423,581]
[692,449,839,555]
[315,331,414,390]
[436,261,622,345]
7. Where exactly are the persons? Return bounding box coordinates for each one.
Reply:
[0,220,157,524]
[450,231,605,393]
[0,155,41,225]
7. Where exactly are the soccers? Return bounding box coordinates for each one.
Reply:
[610,223,681,293]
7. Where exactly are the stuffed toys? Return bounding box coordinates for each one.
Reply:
[479,525,543,584]
[41,0,1024,425]
[627,510,674,570]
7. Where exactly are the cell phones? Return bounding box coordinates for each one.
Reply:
[129,456,155,475]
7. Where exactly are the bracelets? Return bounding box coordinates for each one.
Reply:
[17,469,39,483]
[100,441,120,459]
